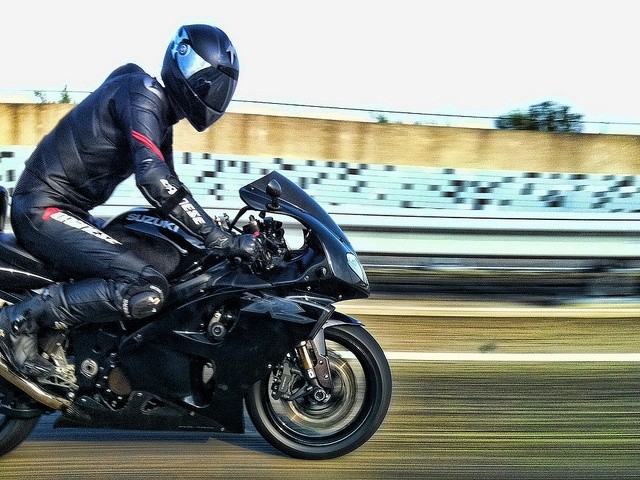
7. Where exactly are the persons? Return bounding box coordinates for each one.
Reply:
[0,25,265,377]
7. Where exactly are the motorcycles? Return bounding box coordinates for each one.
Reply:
[0,171,392,460]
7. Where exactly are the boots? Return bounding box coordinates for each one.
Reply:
[0,282,73,379]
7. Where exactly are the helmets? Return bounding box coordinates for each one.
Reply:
[160,24,239,132]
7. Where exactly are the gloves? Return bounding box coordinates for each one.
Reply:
[206,226,259,258]
